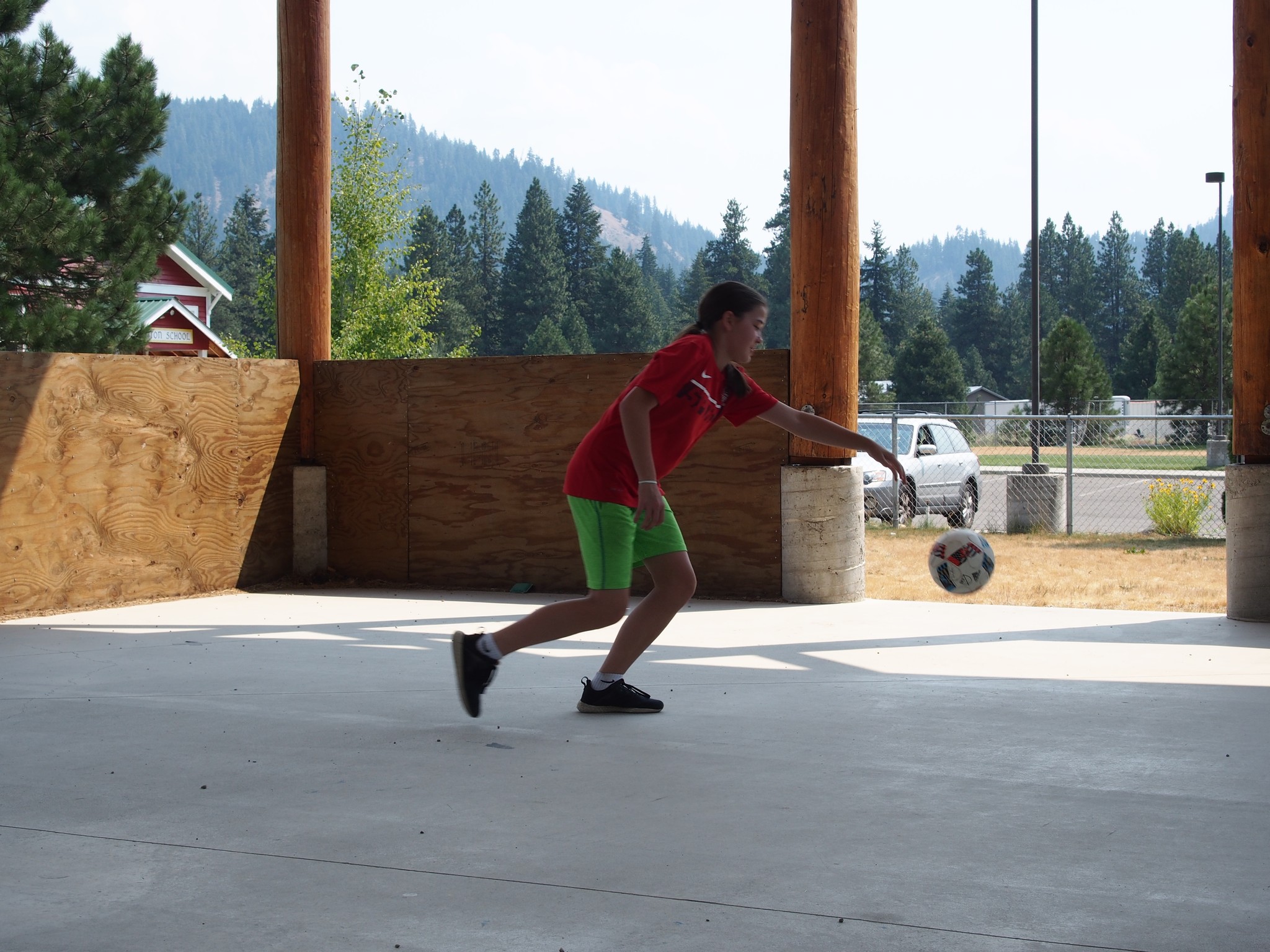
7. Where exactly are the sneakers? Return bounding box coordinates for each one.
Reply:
[577,676,664,713]
[453,631,500,718]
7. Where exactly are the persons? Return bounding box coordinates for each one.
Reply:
[450,280,906,719]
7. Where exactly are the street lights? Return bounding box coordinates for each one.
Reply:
[1204,172,1227,435]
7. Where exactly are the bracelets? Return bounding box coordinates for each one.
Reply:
[638,480,658,484]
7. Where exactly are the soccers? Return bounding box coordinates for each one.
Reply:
[928,527,995,594]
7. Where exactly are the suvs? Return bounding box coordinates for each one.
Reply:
[852,411,983,528]
